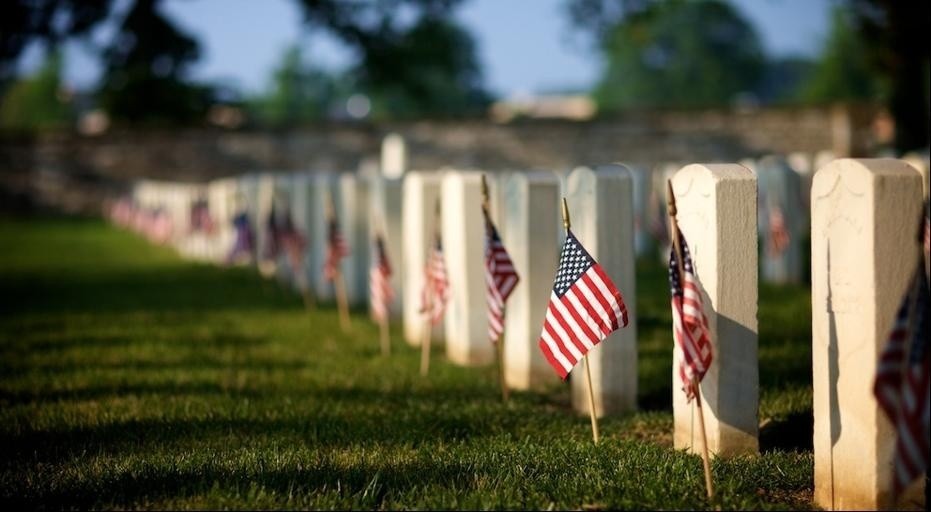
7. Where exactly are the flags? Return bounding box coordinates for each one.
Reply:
[481,215,521,343]
[667,227,713,403]
[418,223,454,323]
[278,218,304,273]
[322,218,350,282]
[540,230,629,382]
[98,185,217,239]
[261,210,282,260]
[366,234,393,323]
[872,258,930,499]
[228,211,256,259]
